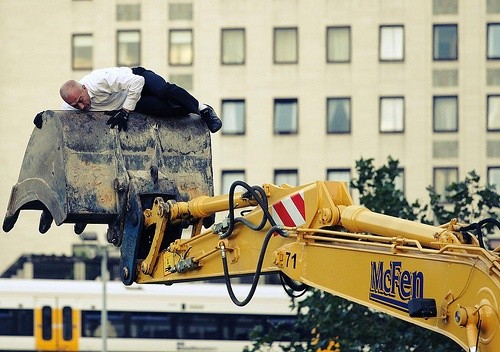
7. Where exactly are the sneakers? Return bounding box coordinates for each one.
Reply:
[199,103,221,132]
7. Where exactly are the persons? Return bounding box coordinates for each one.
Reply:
[59,66,223,134]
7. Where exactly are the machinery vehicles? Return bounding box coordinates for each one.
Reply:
[3,105,500,351]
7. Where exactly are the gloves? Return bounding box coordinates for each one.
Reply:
[103,108,130,130]
